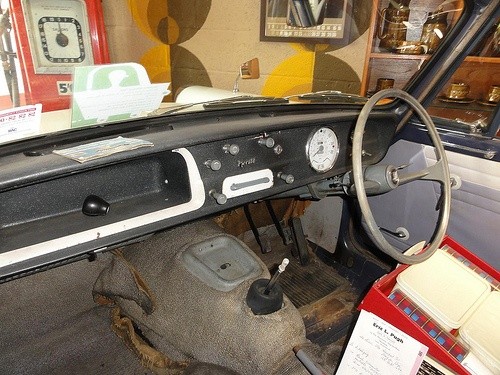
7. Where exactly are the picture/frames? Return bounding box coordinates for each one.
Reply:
[259,0,353,46]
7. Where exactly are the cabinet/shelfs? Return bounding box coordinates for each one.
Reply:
[360,0,500,137]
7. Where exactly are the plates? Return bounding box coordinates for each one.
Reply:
[477,101,500,106]
[439,96,476,104]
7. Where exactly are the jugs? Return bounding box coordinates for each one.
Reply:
[420,12,449,51]
[377,0,412,52]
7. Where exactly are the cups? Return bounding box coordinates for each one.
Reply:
[447,84,470,99]
[487,85,500,103]
[375,78,394,95]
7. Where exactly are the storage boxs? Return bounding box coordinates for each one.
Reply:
[357,236,500,375]
